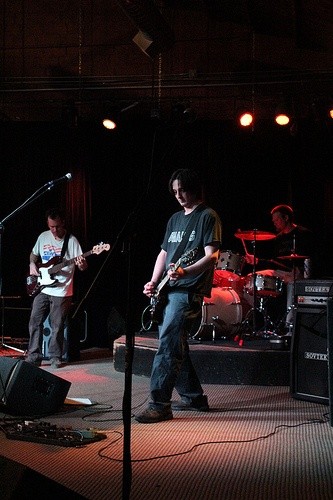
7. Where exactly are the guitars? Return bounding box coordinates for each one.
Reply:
[26,242,111,297]
[150,247,199,323]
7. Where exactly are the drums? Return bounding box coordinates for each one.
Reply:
[215,250,245,282]
[244,273,284,297]
[185,286,242,341]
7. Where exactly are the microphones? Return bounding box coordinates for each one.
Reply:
[44,172,72,186]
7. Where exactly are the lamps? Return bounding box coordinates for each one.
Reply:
[171,98,197,124]
[271,94,295,128]
[62,97,77,118]
[98,91,127,137]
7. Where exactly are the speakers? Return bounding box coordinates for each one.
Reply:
[289,304,333,403]
[0,356,71,417]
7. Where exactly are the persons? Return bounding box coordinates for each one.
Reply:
[24,208,87,368]
[244,205,309,328]
[136,168,222,424]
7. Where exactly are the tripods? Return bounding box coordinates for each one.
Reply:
[233,240,276,336]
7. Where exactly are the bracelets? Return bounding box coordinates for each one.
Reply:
[182,270,187,278]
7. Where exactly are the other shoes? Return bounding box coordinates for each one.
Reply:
[50,364,60,368]
[171,395,210,412]
[24,360,41,366]
[134,403,173,422]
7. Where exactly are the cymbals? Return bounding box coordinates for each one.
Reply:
[234,231,276,240]
[276,255,309,258]
